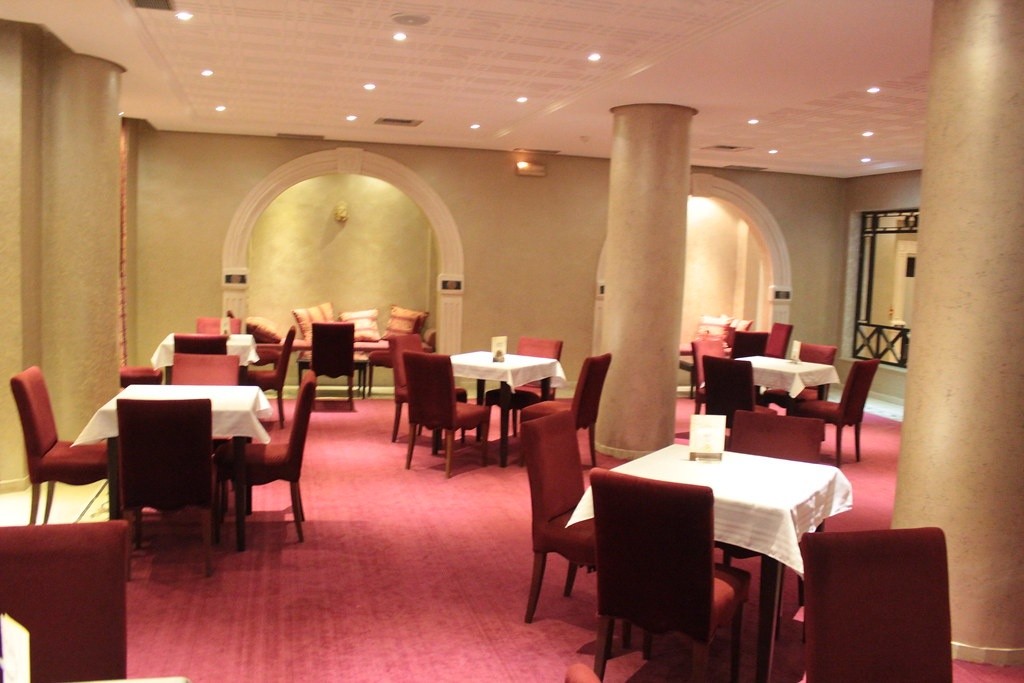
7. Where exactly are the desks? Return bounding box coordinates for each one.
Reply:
[701,355,839,420]
[149,331,256,387]
[73,383,272,558]
[444,345,571,477]
[563,445,853,683]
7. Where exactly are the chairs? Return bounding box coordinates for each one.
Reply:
[0,316,956,683]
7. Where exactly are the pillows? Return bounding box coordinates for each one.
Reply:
[294,300,335,344]
[693,314,730,343]
[250,317,281,345]
[342,306,382,346]
[734,318,755,333]
[377,306,433,340]
